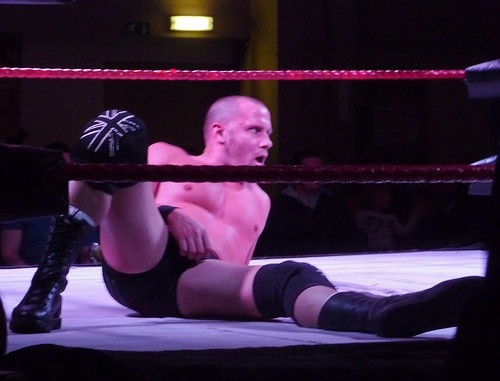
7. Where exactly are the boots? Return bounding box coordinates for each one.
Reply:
[316,276,487,336]
[9,215,96,334]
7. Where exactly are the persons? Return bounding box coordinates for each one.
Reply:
[8,94,486,339]
[0,143,486,267]
